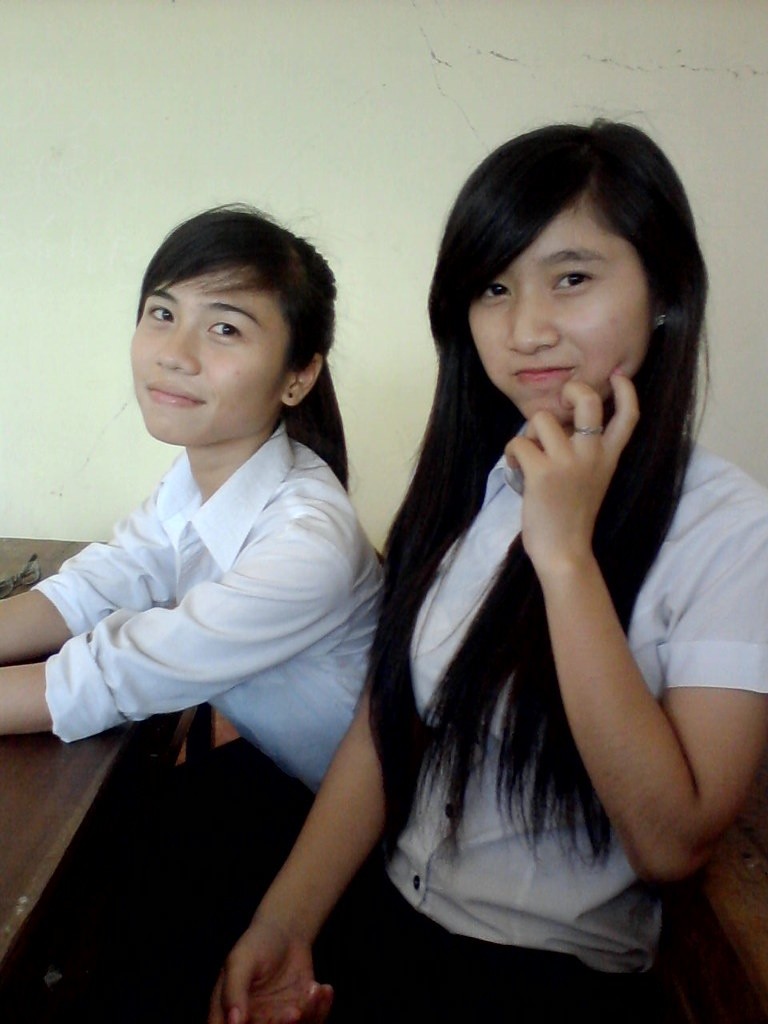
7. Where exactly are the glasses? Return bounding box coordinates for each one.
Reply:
[0,554,42,599]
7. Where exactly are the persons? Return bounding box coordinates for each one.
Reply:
[212,115,767,1024]
[0,201,383,1023]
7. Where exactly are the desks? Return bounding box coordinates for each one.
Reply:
[0,559,216,992]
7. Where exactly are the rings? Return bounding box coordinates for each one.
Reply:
[571,426,603,437]
